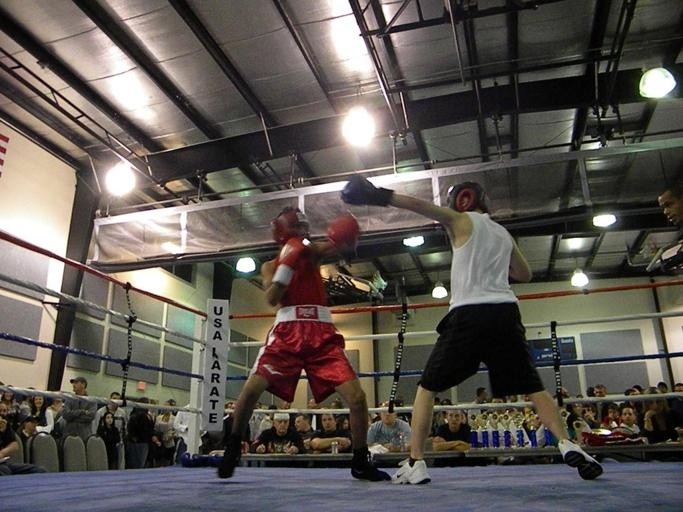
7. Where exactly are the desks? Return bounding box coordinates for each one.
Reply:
[202,446,464,468]
[467,442,682,463]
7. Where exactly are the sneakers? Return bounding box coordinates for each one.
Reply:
[216,447,242,478]
[350,463,392,482]
[558,438,604,481]
[392,458,432,484]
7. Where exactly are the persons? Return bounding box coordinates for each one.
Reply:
[224,401,412,468]
[427,382,683,468]
[216,205,392,482]
[1,376,203,477]
[655,182,682,227]
[337,171,605,486]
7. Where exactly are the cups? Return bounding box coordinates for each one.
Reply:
[331,441,339,454]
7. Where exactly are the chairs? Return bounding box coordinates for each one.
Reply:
[23,436,34,465]
[62,432,87,472]
[30,431,59,473]
[8,431,25,465]
[85,434,109,471]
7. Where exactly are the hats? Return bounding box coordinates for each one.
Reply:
[273,413,290,420]
[21,415,40,422]
[70,376,88,387]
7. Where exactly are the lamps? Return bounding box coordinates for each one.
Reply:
[340,84,377,149]
[569,257,590,288]
[431,271,449,300]
[637,61,678,100]
